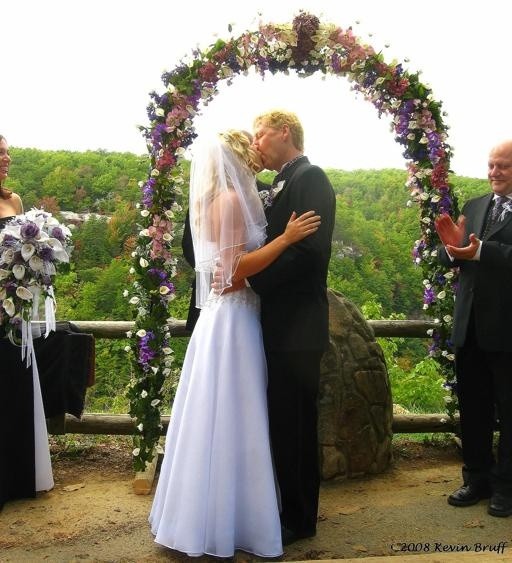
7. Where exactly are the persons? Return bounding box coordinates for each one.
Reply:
[0,135,55,496]
[251,109,337,544]
[432,139,512,518]
[148,129,324,559]
[181,179,272,334]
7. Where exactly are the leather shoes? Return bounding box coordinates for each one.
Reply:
[447,481,489,506]
[488,490,512,517]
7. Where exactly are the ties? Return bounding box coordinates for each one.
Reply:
[491,196,507,224]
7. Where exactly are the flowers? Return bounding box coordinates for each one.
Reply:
[122,7,462,473]
[257,187,282,211]
[0,204,75,344]
